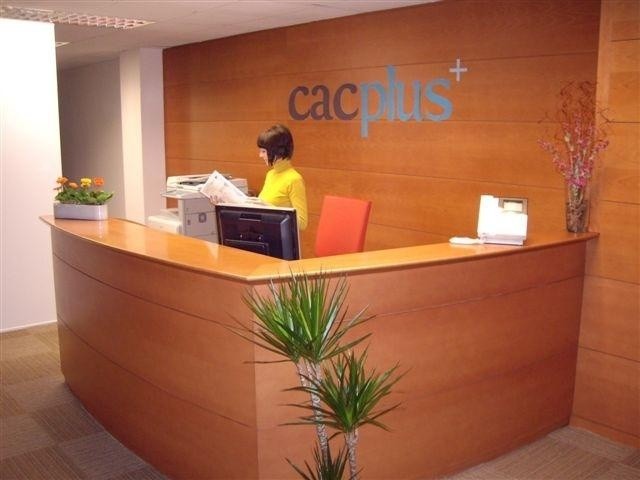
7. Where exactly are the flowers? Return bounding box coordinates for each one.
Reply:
[52,177,113,205]
[536,77,615,201]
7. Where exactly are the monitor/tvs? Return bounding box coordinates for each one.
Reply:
[214,203,301,261]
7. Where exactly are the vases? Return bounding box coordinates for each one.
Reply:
[54,203,108,220]
[564,181,592,233]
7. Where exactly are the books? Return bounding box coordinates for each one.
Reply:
[202,171,249,206]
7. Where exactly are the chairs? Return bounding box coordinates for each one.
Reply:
[313,195,373,257]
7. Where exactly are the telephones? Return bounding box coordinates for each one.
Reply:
[476,194,528,244]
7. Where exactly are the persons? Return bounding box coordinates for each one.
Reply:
[244,124,308,231]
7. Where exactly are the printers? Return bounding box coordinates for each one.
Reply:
[146,174,248,243]
